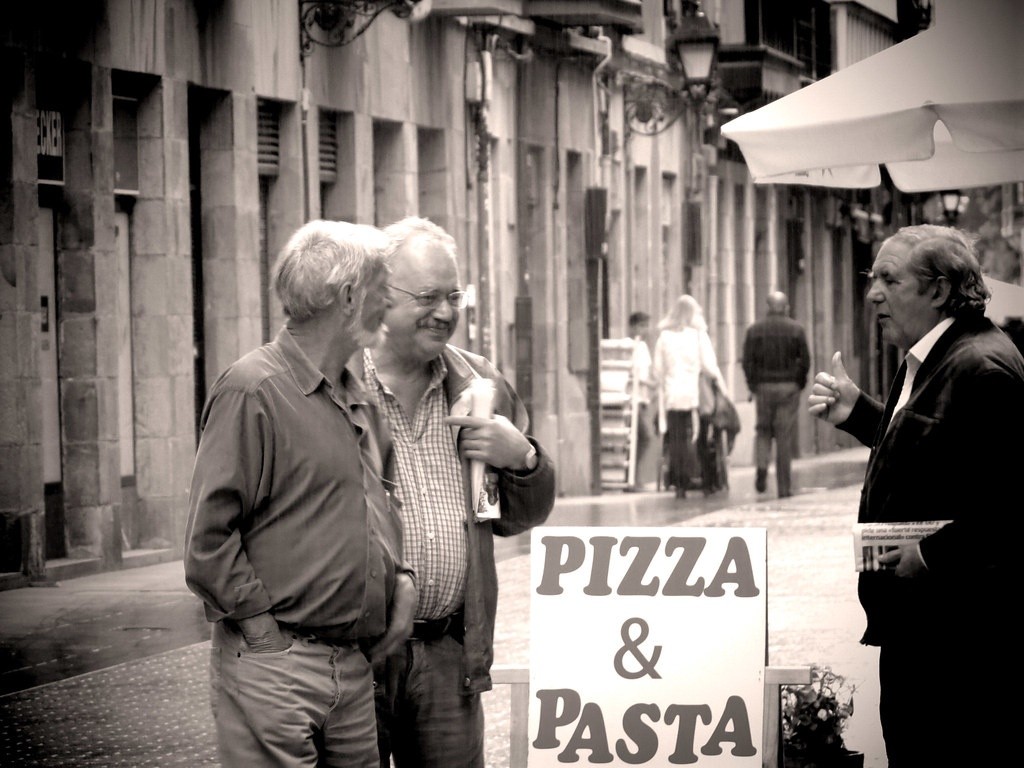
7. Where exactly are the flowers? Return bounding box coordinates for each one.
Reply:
[779,666,856,753]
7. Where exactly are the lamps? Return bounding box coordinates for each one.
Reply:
[623,0,723,136]
[940,187,967,225]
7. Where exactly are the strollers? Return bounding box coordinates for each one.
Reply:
[658,372,741,493]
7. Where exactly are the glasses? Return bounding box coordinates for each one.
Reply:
[388,283,468,310]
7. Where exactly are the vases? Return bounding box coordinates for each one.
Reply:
[781,748,866,768]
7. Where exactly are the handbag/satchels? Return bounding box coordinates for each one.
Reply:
[698,371,741,433]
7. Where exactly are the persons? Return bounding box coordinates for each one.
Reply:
[743,291,810,498]
[483,473,499,505]
[811,223,1023,767]
[345,214,555,768]
[624,312,652,492]
[656,294,741,499]
[183,219,405,767]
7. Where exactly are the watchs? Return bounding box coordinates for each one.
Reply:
[523,443,538,471]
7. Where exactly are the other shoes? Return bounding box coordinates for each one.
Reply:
[756,469,768,493]
[703,482,721,494]
[677,488,685,498]
[779,490,794,498]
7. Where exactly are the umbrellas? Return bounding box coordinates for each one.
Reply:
[720,0,1023,193]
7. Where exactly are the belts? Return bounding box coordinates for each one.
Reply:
[406,606,466,643]
[292,624,358,647]
